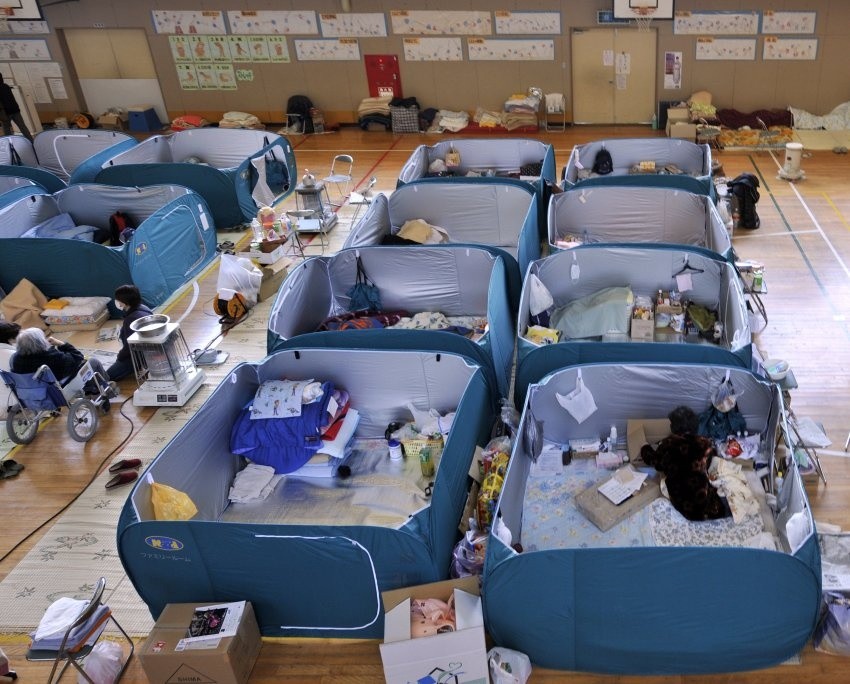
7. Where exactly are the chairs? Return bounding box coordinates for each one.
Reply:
[544,93,566,133]
[26,578,134,684]
[284,96,314,135]
[286,154,377,262]
[754,117,780,158]
[774,412,826,484]
[699,118,723,153]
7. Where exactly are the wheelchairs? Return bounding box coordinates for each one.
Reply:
[0,359,119,445]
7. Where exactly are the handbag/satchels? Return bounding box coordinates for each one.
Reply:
[455,530,490,574]
[217,254,264,304]
[555,376,598,424]
[147,473,199,520]
[263,136,289,186]
[446,148,461,166]
[486,646,532,684]
[525,410,543,464]
[345,256,383,313]
[810,591,850,656]
[710,379,744,413]
[529,274,554,316]
[730,325,750,352]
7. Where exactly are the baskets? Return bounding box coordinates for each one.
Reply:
[399,422,443,456]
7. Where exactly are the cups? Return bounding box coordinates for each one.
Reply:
[384,422,402,439]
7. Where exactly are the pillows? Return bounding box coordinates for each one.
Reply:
[40,296,111,324]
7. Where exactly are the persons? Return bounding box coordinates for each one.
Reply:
[106,286,152,380]
[640,405,725,521]
[9,327,118,397]
[0,73,33,144]
[0,321,21,418]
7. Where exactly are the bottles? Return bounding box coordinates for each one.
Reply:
[252,218,261,240]
[560,445,571,465]
[753,270,763,292]
[733,209,739,227]
[387,439,401,460]
[735,430,748,441]
[606,438,612,453]
[657,289,663,304]
[774,472,784,494]
[419,445,434,477]
[652,114,657,129]
[583,231,588,244]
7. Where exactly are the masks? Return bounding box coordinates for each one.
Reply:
[115,300,126,311]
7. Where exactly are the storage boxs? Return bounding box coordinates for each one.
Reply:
[402,438,443,456]
[236,230,292,302]
[665,109,716,144]
[96,105,156,132]
[801,471,819,493]
[139,602,263,684]
[631,291,700,339]
[368,121,386,131]
[379,574,491,684]
[49,312,109,331]
[570,420,670,532]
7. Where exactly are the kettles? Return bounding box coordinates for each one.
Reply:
[301,169,316,186]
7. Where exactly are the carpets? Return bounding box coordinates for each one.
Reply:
[0,190,393,637]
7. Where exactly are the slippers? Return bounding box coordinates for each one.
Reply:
[105,472,138,487]
[0,469,19,478]
[109,458,141,471]
[0,460,24,470]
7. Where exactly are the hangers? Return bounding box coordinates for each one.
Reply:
[672,259,704,278]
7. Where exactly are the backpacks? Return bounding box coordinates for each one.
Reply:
[727,173,761,229]
[109,210,132,247]
[591,147,613,175]
[287,95,315,134]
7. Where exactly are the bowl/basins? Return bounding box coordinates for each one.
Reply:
[129,315,169,338]
[762,359,790,379]
